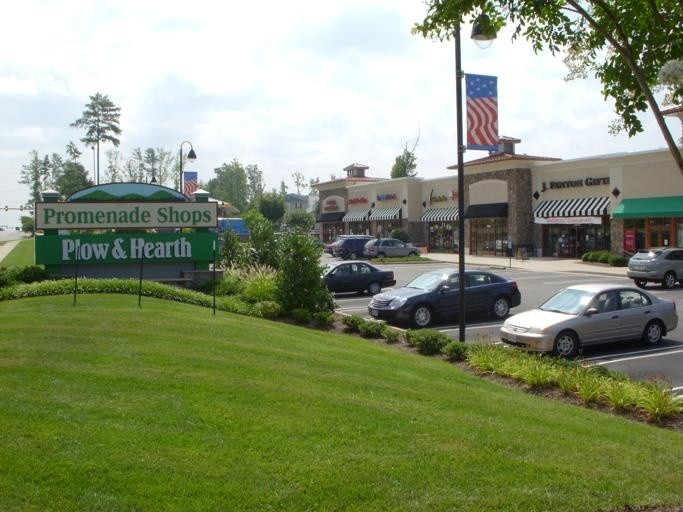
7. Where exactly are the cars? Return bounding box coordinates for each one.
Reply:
[320,260,396,295]
[362,238,420,258]
[331,235,375,260]
[499,281,678,362]
[367,269,520,333]
[323,243,332,253]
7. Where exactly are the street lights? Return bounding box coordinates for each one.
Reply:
[179,141,196,194]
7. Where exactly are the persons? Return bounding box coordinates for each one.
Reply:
[557,235,568,256]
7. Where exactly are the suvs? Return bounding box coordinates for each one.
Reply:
[626,246,682,289]
